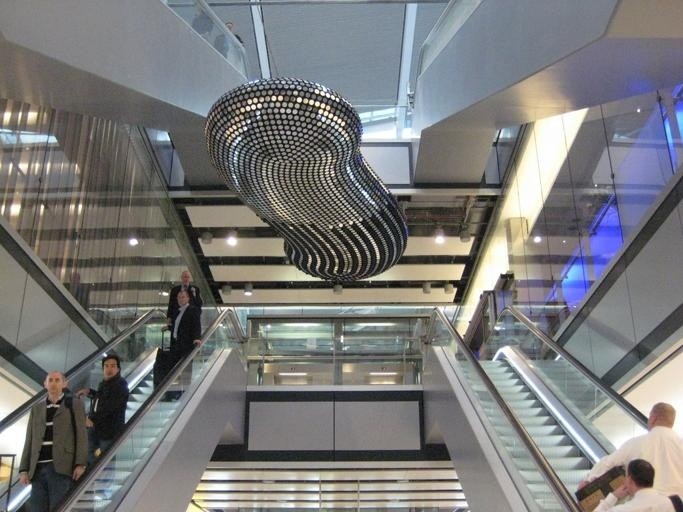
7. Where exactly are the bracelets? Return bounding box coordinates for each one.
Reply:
[78,464,86,469]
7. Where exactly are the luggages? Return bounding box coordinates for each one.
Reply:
[151,347,185,403]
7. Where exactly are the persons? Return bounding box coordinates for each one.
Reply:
[160,290,205,405]
[590,457,678,511]
[166,271,201,327]
[62,273,90,314]
[74,354,128,499]
[19,370,87,511]
[578,402,683,511]
